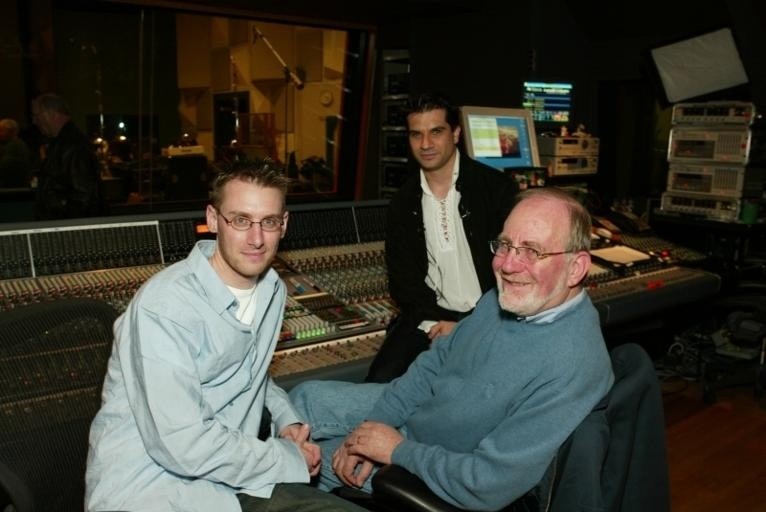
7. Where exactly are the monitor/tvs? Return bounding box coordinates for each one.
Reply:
[522,80,573,124]
[459,106,541,172]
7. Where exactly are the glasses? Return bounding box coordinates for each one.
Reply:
[216,209,284,232]
[489,240,574,265]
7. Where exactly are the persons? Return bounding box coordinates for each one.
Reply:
[83,159,322,511]
[361,92,525,383]
[0,117,33,188]
[30,92,106,221]
[273,188,616,510]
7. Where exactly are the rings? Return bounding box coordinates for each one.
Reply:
[356,435,362,445]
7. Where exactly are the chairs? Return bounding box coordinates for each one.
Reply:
[371,343,657,509]
